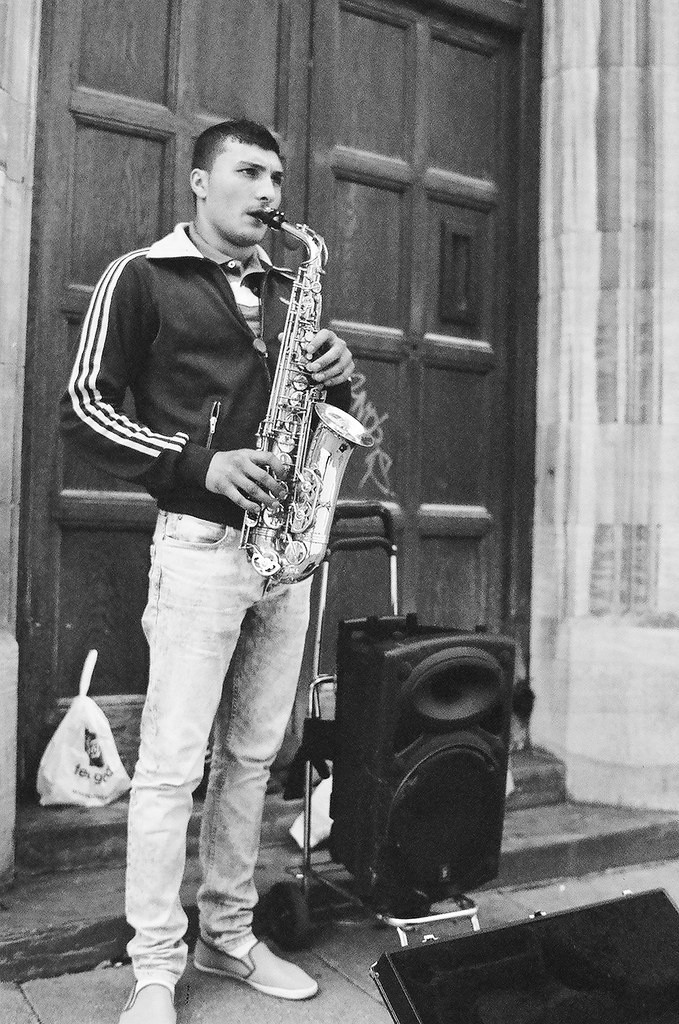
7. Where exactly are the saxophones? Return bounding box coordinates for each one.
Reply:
[238,210,376,586]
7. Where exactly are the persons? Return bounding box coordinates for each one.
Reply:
[55,108,357,1024]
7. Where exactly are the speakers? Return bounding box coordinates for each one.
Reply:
[327,610,517,913]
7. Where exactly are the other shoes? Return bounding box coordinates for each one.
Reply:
[193,936,318,999]
[119,980,176,1024]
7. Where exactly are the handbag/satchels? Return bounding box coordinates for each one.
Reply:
[37,649,130,808]
